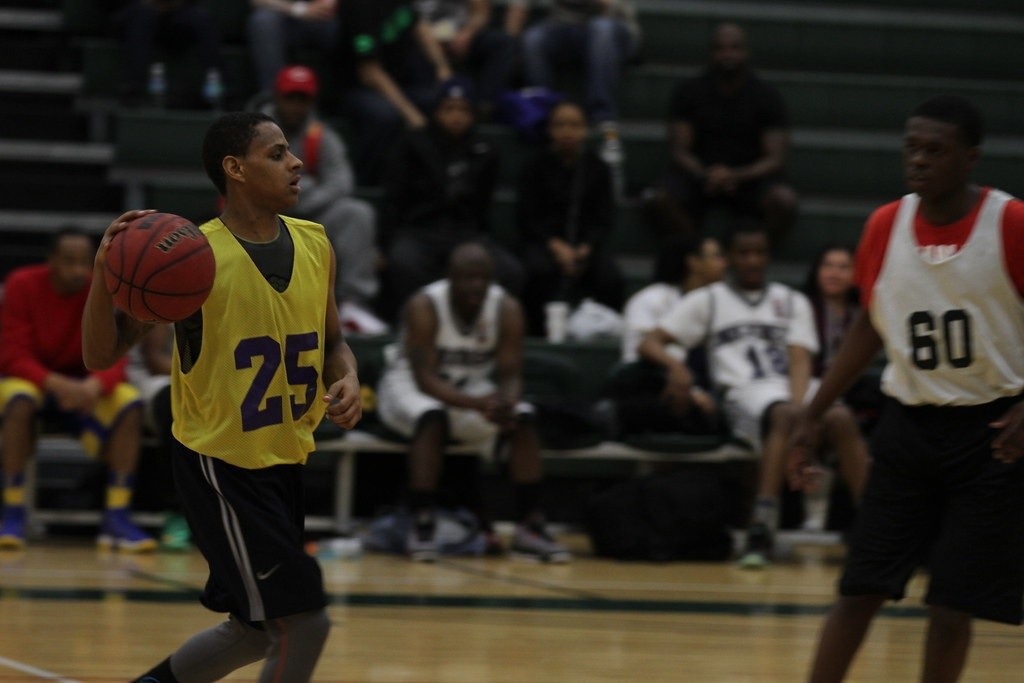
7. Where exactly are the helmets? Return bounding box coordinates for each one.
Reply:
[274,67,317,97]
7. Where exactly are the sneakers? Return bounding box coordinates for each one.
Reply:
[99,512,157,552]
[0,501,27,547]
[407,519,442,562]
[510,524,572,564]
[744,525,773,566]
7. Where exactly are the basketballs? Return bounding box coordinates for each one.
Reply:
[102,213,216,325]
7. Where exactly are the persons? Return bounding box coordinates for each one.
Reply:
[0,227,160,551]
[126,323,193,550]
[530,221,887,564]
[372,242,584,560]
[783,94,1023,683]
[131,1,799,337]
[81,108,362,683]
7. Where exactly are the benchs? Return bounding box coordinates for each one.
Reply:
[0,0,1024,542]
